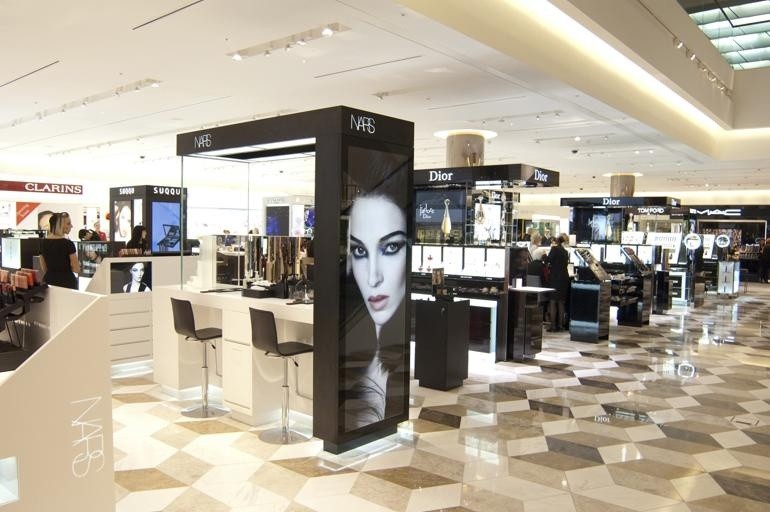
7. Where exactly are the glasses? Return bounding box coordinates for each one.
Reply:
[61,212,68,216]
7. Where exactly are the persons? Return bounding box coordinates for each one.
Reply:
[339,160,405,431]
[40,212,80,289]
[523,227,571,332]
[84,243,106,277]
[123,262,151,293]
[248,228,259,233]
[511,249,527,286]
[115,200,131,244]
[758,238,770,283]
[79,228,101,241]
[127,226,149,254]
[38,211,54,230]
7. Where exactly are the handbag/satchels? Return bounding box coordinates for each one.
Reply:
[528,260,550,283]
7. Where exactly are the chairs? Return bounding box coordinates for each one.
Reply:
[170,297,230,419]
[249,306,315,445]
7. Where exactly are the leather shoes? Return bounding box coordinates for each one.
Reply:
[547,326,563,332]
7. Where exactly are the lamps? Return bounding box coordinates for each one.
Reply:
[231,26,334,62]
[10,86,143,129]
[673,37,726,90]
